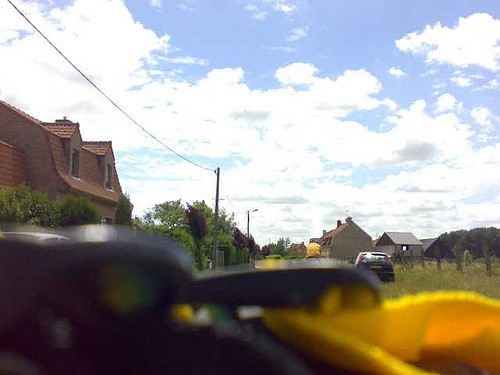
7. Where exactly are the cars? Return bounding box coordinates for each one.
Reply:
[355,251,395,283]
